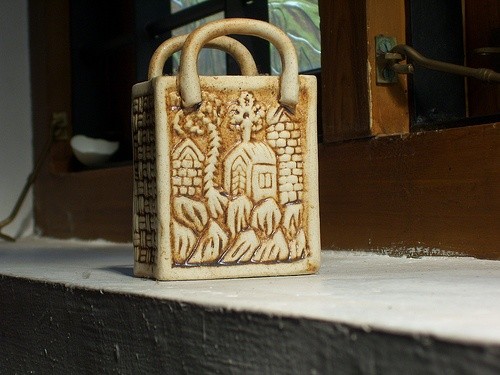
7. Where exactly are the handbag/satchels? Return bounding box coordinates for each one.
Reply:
[129,16,325,281]
